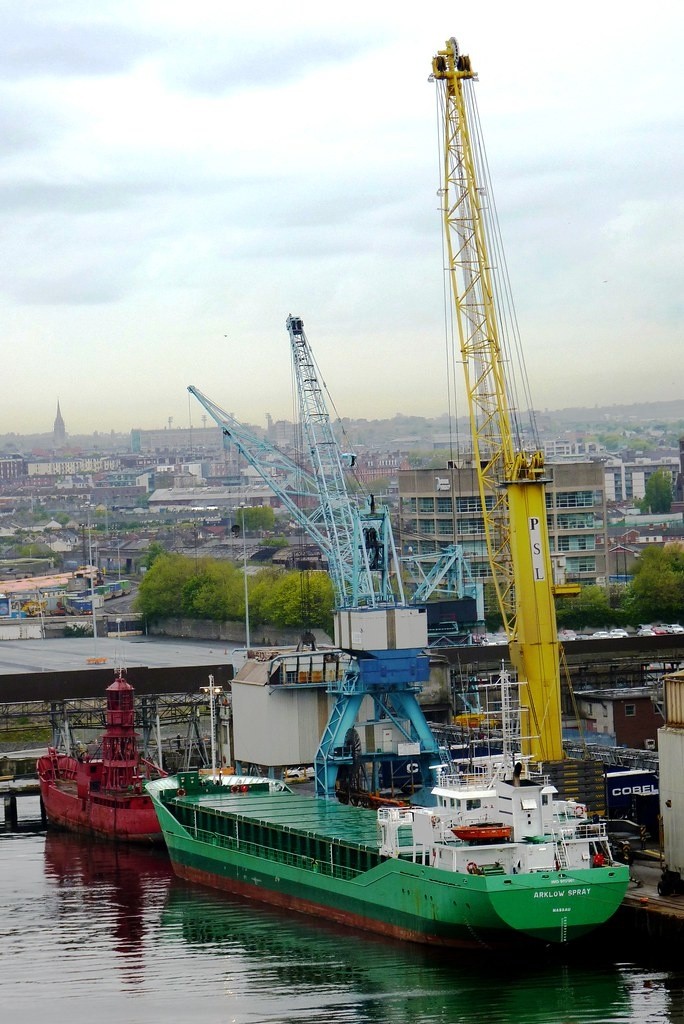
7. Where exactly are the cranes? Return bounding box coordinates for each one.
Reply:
[185,314,479,798]
[429,36,569,790]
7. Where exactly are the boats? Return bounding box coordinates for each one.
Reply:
[154,874,635,1024]
[146,657,632,948]
[35,618,178,844]
[37,826,172,962]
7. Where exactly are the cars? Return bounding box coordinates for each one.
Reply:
[471,623,684,646]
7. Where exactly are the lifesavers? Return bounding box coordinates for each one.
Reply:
[177,788,186,796]
[230,785,240,793]
[466,861,478,872]
[240,784,248,792]
